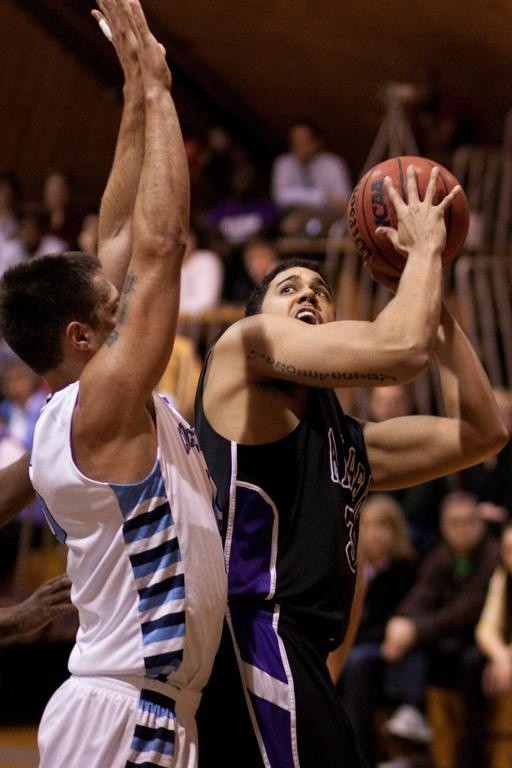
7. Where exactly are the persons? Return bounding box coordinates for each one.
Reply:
[195,163,510,768]
[326,382,512,768]
[2,128,223,639]
[1,0,231,767]
[217,118,484,286]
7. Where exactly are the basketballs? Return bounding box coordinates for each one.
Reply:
[347,155,470,278]
